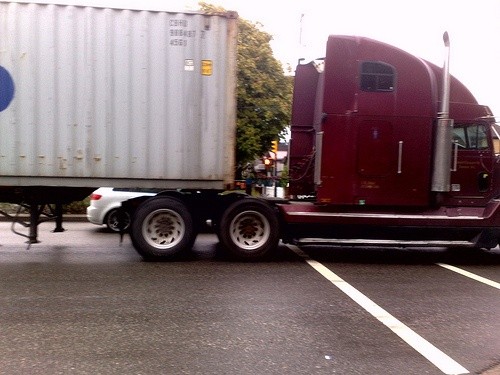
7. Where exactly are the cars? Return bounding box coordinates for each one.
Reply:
[85,187,201,234]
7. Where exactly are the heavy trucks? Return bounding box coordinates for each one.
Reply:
[0,0,500,263]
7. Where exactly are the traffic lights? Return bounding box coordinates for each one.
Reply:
[271,140,279,154]
[264,158,274,168]
